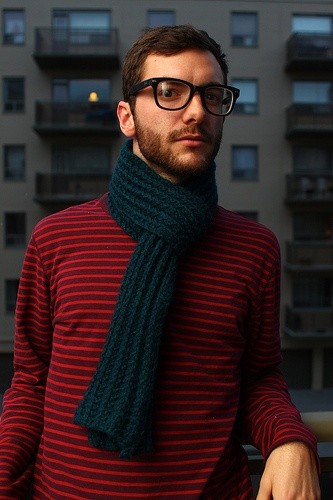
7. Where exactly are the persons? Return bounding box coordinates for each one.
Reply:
[1,24,323,500]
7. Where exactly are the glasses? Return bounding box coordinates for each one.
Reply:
[123,77,240,117]
